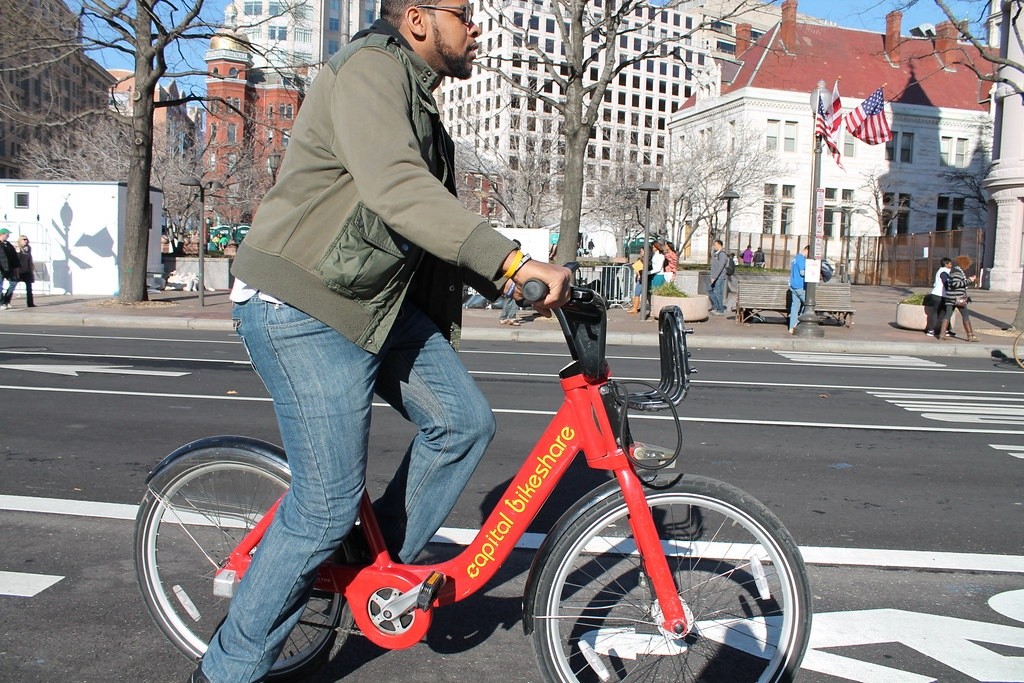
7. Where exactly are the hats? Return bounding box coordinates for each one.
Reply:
[0,228,12,234]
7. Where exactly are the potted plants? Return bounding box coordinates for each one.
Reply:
[895,295,956,329]
[650,282,711,322]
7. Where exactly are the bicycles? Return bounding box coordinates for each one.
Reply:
[132,274,814,683]
[1011,329,1024,370]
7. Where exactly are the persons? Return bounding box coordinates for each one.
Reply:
[743,245,753,266]
[789,245,809,333]
[588,239,594,256]
[499,239,520,325]
[627,246,651,314]
[731,253,739,265]
[212,234,228,251]
[186,0,572,683]
[664,242,677,282]
[6,235,36,307]
[0,228,21,310]
[709,240,728,316]
[753,247,765,268]
[648,241,666,289]
[927,255,979,342]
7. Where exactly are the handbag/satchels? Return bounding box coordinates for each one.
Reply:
[955,297,967,308]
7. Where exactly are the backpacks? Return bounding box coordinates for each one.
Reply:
[717,251,734,277]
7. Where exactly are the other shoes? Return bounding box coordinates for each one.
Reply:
[926,330,938,337]
[335,527,367,567]
[788,329,795,333]
[946,331,956,337]
[711,310,725,316]
[5,302,12,308]
[28,304,36,307]
[508,320,520,326]
[0,303,6,310]
[500,317,508,324]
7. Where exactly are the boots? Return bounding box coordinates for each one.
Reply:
[939,319,952,340]
[627,296,640,314]
[964,321,980,341]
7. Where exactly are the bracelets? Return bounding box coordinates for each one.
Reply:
[503,250,532,279]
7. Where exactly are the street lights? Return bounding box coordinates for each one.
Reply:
[267,147,282,188]
[793,78,833,337]
[636,181,660,321]
[178,175,226,308]
[719,187,740,302]
[486,201,494,224]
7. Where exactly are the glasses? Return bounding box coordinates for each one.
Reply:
[413,5,473,25]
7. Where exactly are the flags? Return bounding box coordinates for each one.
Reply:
[818,80,893,170]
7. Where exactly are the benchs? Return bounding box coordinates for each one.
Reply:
[737,282,857,323]
